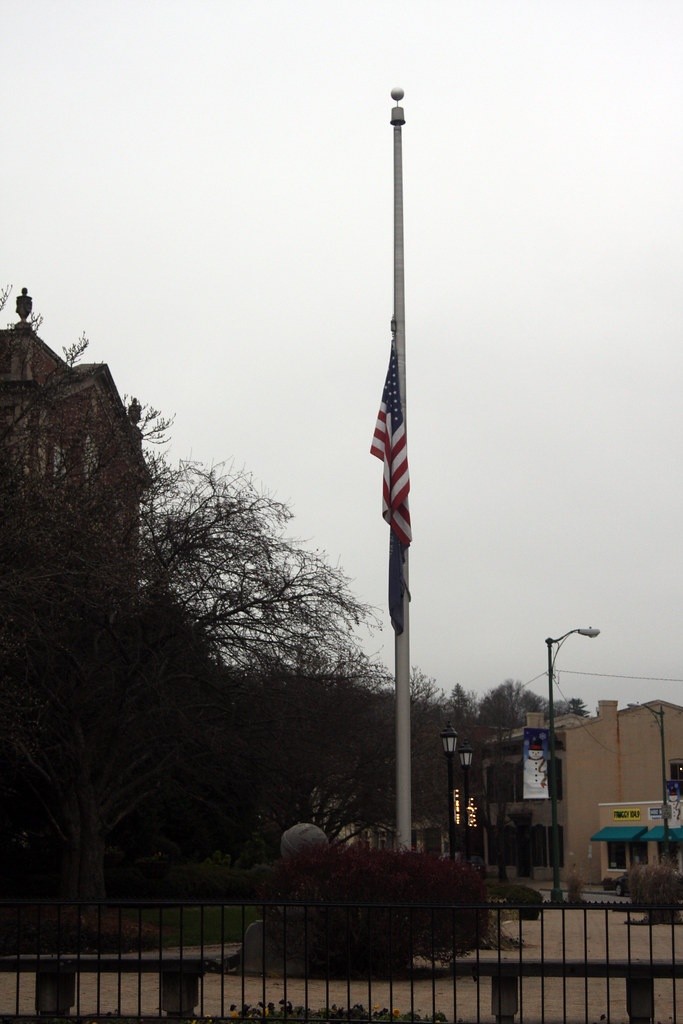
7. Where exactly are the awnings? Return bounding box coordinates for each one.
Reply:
[590,826,648,841]
[639,825,683,842]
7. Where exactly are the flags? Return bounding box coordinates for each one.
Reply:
[389,526,407,635]
[371,341,412,545]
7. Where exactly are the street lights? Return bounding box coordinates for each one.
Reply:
[441,720,463,862]
[628,700,671,862]
[543,622,602,899]
[460,742,472,866]
